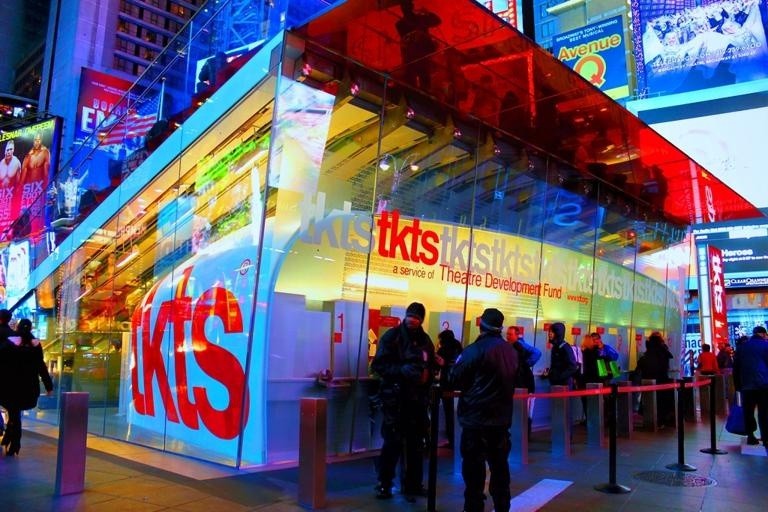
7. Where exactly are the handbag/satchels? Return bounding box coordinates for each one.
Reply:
[595,359,620,378]
[725,406,756,436]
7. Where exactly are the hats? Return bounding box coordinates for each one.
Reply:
[405,303,424,323]
[479,309,503,333]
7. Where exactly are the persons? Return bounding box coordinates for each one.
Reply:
[20,133,50,244]
[395,0,441,96]
[0,309,14,436]
[197,51,229,94]
[0,140,21,242]
[370,302,435,499]
[445,47,667,215]
[447,309,522,512]
[0,319,54,456]
[52,167,89,218]
